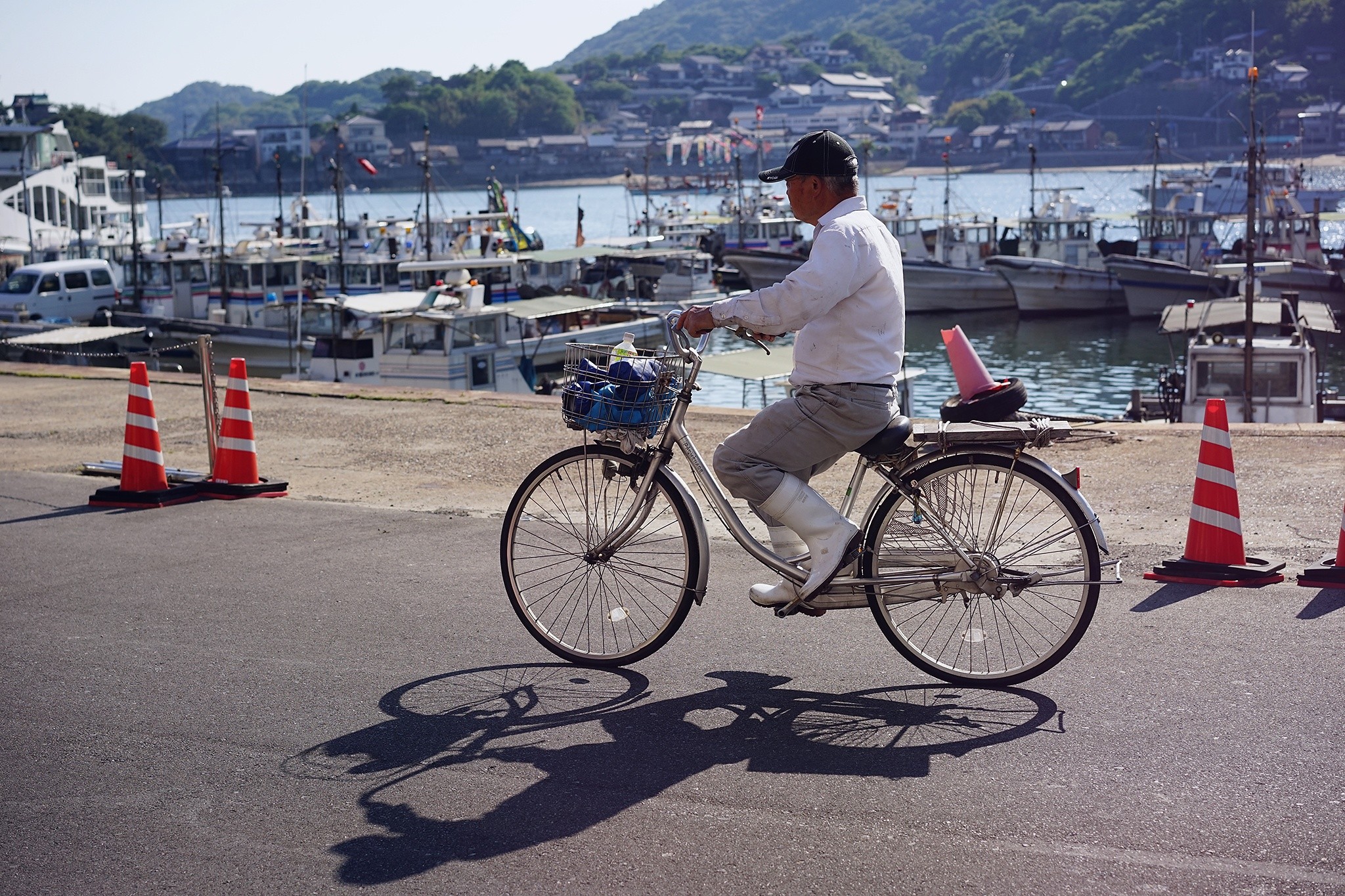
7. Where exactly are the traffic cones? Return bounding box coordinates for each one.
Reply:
[86,361,186,510]
[180,358,289,498]
[1296,509,1344,585]
[1147,399,1286,588]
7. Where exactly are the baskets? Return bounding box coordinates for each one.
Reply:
[559,342,683,439]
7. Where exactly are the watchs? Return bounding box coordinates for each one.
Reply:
[709,306,713,313]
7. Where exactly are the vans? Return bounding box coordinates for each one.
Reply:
[1,259,122,325]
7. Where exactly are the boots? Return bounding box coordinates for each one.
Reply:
[758,473,864,604]
[748,525,827,617]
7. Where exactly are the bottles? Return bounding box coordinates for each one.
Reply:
[608,332,638,374]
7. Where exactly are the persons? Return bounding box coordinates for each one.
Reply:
[676,129,905,618]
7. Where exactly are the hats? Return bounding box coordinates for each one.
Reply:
[757,129,859,184]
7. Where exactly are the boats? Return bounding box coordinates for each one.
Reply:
[55,210,754,416]
[1123,294,1343,429]
[667,173,1345,318]
[0,92,152,262]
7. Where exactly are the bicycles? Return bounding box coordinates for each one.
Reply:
[498,309,1124,688]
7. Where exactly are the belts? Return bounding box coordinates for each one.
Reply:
[838,382,893,389]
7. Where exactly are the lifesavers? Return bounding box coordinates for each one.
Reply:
[558,285,579,296]
[617,281,628,298]
[600,282,613,296]
[518,285,536,300]
[536,285,556,298]
[939,378,1028,423]
[640,279,654,298]
